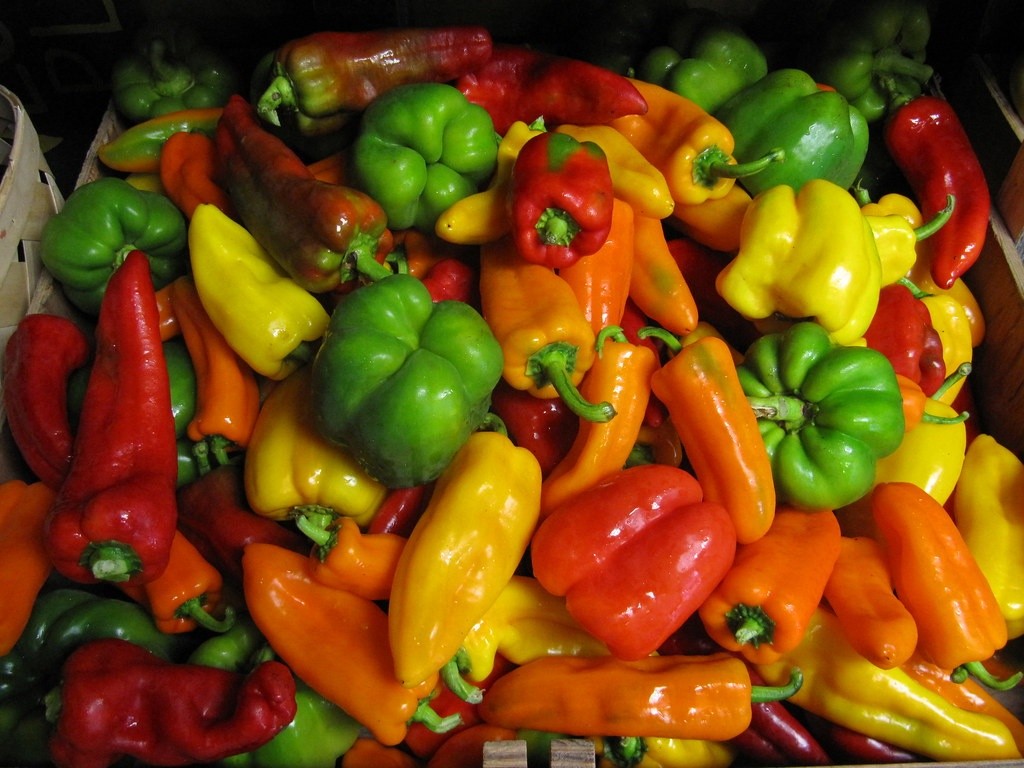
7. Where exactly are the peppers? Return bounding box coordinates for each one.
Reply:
[1,1,1024,768]
